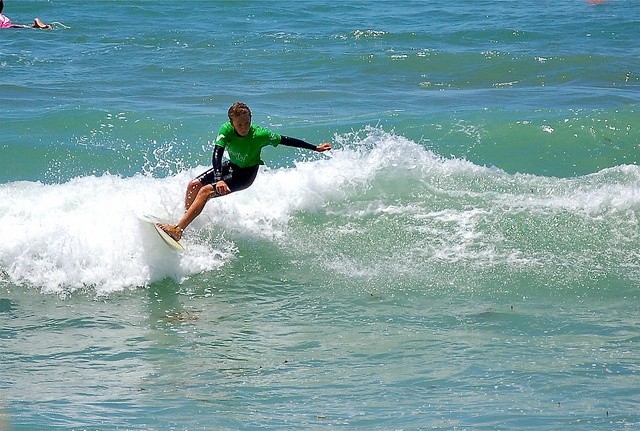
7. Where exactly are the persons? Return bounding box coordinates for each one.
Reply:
[0,0,52,30]
[157,102,332,241]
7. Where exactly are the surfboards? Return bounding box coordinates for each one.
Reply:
[142,213,187,255]
